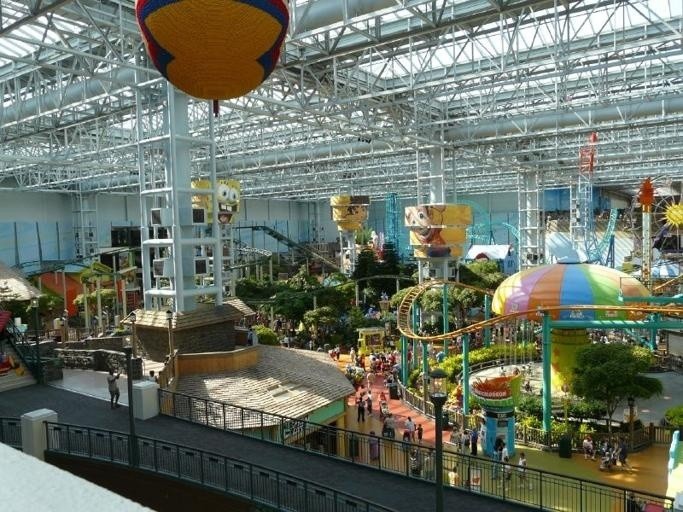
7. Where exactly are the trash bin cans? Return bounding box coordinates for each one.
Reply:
[560,439,571,458]
[441,412,448,430]
[390,384,397,399]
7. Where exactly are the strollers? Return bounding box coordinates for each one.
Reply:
[381,424,391,437]
[379,400,389,417]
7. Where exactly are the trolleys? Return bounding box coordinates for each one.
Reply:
[599,452,617,471]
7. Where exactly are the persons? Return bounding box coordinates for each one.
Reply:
[147,370,157,383]
[106,367,121,409]
[255,290,673,489]
[624,493,645,512]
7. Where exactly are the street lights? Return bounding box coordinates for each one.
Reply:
[427,369,447,512]
[165,309,174,357]
[30,295,42,385]
[130,312,137,357]
[120,330,140,467]
[63,309,68,341]
